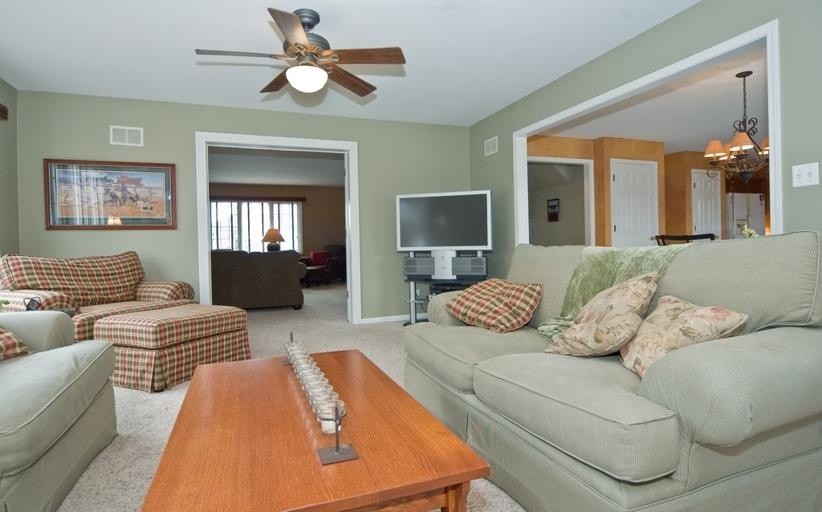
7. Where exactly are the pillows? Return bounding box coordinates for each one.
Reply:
[0,324,34,364]
[541,266,663,361]
[614,293,753,386]
[308,248,330,266]
[442,274,545,336]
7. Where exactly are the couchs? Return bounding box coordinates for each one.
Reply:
[400,224,822,510]
[0,248,200,341]
[211,247,311,312]
[298,243,347,286]
[93,297,252,394]
[1,305,124,512]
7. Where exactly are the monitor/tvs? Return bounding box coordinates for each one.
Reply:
[396,189,493,256]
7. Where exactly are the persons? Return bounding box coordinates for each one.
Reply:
[104,184,138,206]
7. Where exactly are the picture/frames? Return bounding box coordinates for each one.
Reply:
[41,156,178,234]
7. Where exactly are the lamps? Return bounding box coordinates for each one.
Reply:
[281,48,331,97]
[700,64,772,182]
[260,226,287,252]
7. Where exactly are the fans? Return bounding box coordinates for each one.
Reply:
[192,0,412,112]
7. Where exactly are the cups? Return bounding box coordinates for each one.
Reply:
[281,335,345,435]
